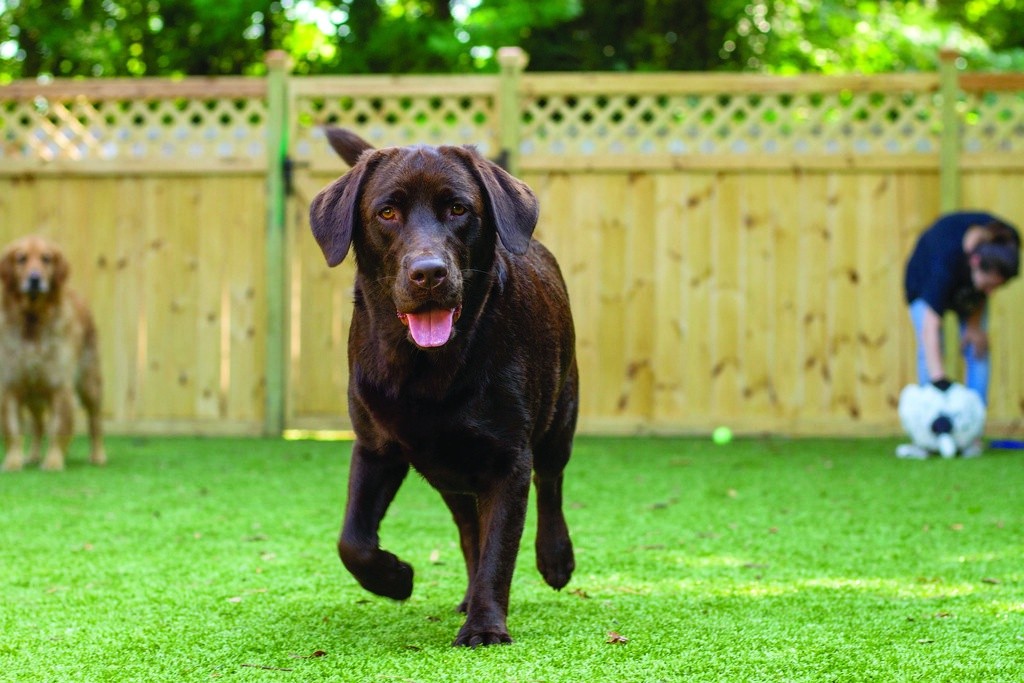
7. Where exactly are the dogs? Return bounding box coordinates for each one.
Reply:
[305,118,583,651]
[0,233,111,475]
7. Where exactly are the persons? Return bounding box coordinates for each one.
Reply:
[904,213,1020,446]
